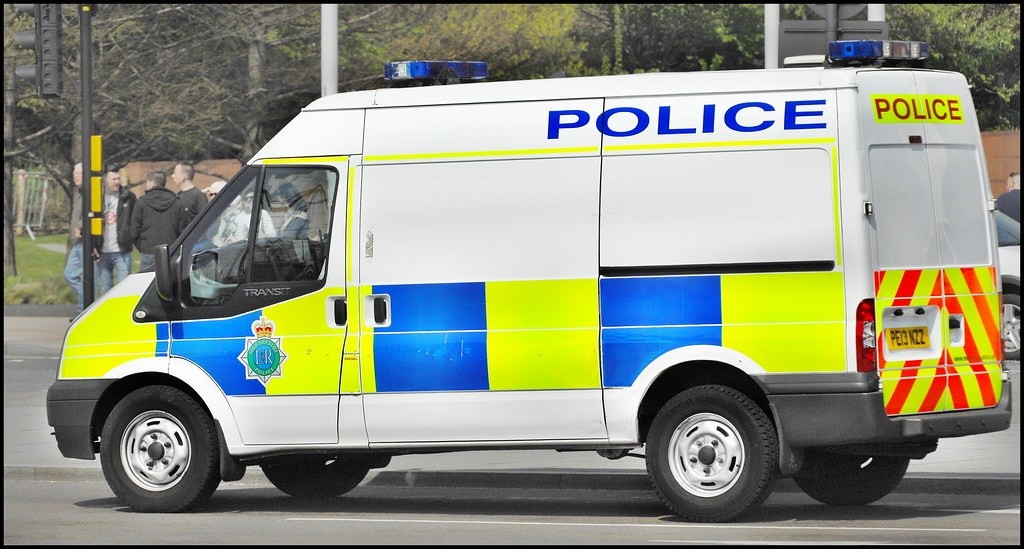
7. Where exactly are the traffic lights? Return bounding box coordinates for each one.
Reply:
[14,3,65,99]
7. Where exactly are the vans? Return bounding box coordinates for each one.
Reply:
[996,211,1021,363]
[41,37,1016,527]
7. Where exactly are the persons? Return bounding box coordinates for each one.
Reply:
[993,169,1022,227]
[62,161,311,322]
